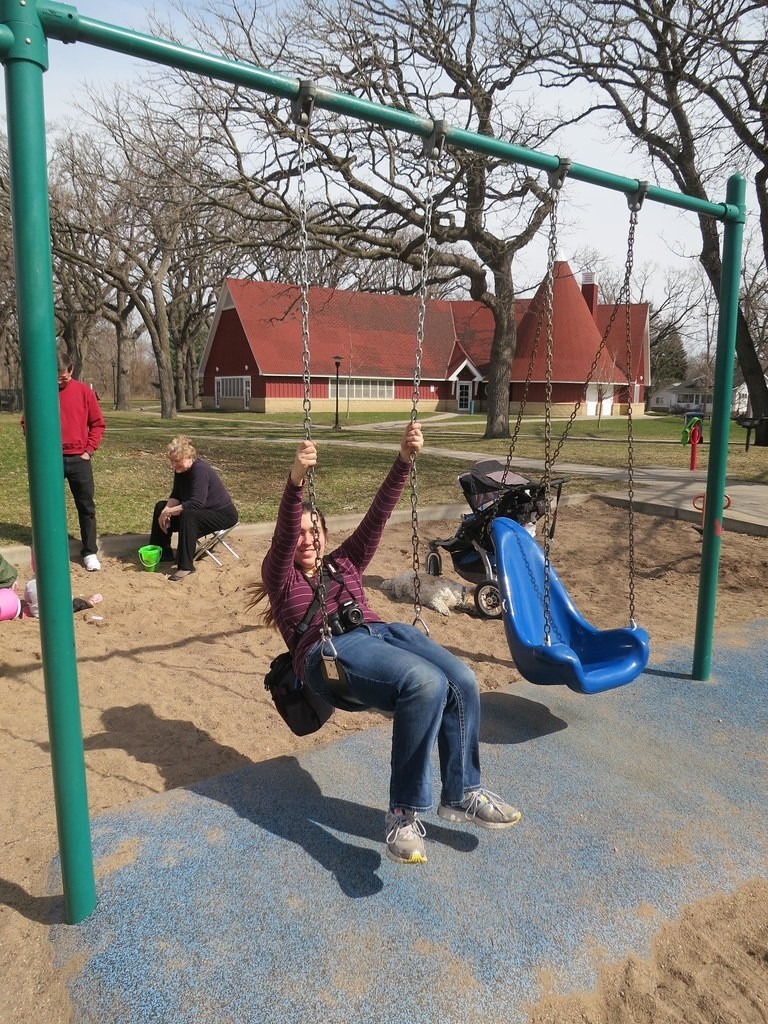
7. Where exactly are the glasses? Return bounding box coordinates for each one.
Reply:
[58,367,68,380]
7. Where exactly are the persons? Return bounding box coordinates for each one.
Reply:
[261,420,521,864]
[21,352,106,572]
[148,436,238,581]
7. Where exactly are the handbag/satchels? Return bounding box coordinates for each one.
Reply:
[263,651,335,737]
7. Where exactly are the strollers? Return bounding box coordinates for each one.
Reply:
[425,460,572,617]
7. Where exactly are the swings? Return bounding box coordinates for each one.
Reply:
[489,170,651,695]
[292,90,446,714]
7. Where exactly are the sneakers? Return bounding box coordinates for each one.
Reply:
[385,806,427,864]
[438,787,521,828]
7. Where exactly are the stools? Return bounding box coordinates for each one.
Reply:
[194,522,240,566]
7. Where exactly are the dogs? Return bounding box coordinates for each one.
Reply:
[380,570,479,617]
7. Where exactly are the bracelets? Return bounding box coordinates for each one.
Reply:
[84,450,93,458]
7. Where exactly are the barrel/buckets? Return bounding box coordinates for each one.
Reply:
[0,588,21,621]
[139,545,162,572]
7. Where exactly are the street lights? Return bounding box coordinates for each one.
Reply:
[332,356,344,429]
[111,360,117,405]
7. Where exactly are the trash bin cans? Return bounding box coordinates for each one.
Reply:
[684,412,704,444]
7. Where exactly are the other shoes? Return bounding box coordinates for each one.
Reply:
[168,567,196,581]
[90,594,102,603]
[84,553,101,571]
